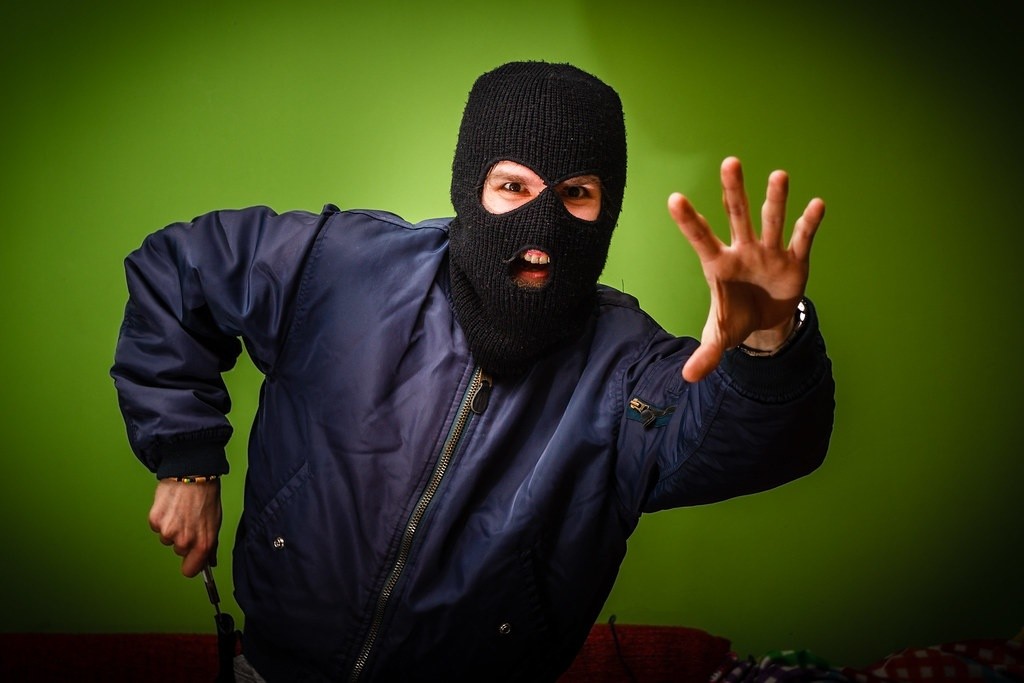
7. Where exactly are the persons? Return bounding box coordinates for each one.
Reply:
[110,58,837,683]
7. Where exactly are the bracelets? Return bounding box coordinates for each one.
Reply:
[170,475,220,484]
[735,298,808,357]
